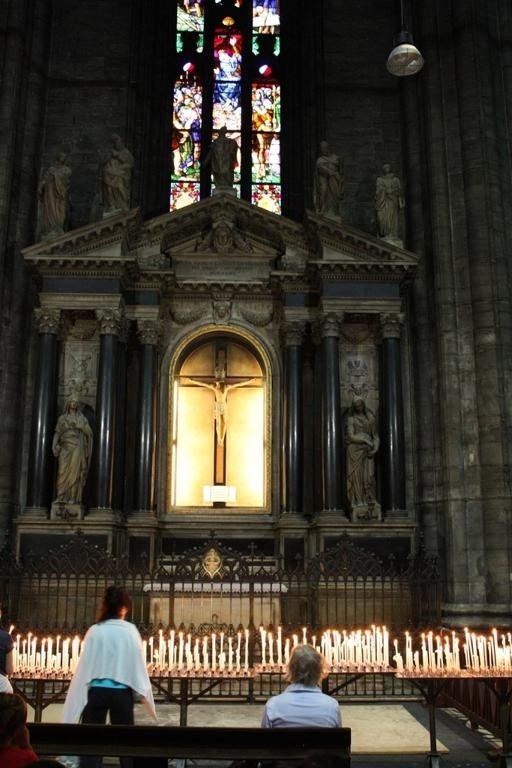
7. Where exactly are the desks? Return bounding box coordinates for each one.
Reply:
[7,672,74,722]
[144,662,253,727]
[255,663,396,698]
[395,668,512,768]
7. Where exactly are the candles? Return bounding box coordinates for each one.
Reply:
[143,626,250,669]
[394,630,460,668]
[463,629,512,666]
[258,627,389,661]
[8,626,84,673]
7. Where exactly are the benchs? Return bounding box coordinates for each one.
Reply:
[25,723,352,768]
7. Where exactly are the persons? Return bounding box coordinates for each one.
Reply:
[0,691,41,768]
[0,599,16,694]
[34,150,75,246]
[198,218,248,255]
[372,162,408,242]
[60,585,162,768]
[212,300,232,327]
[170,0,282,216]
[52,393,95,506]
[95,132,136,219]
[312,140,347,225]
[260,643,344,768]
[343,395,381,512]
